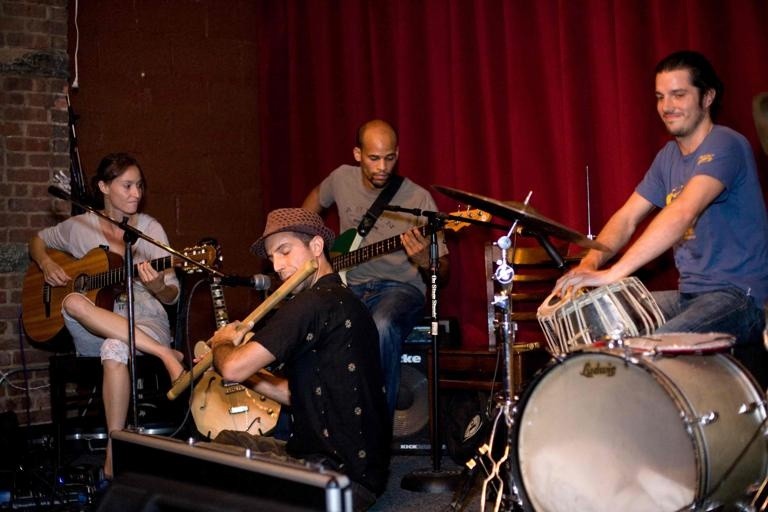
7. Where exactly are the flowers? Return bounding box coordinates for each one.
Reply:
[532,228,565,267]
[206,273,272,291]
[48,184,78,203]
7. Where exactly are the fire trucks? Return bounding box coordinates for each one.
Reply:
[166,258,318,402]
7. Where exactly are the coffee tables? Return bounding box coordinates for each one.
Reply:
[564,249,624,261]
[431,184,613,255]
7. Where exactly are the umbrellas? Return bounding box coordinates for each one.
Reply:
[169,349,185,385]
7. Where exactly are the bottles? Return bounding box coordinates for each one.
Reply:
[250,208,334,258]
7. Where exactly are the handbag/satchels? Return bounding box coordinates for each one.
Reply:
[438,261,441,272]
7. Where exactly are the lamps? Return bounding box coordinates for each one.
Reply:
[48,265,189,485]
[427,241,567,458]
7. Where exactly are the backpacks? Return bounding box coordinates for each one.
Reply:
[508,352,768,512]
[622,330,736,357]
[538,276,667,353]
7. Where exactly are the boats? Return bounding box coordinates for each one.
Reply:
[391,336,433,452]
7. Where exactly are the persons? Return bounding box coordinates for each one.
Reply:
[551,49,768,356]
[193,208,396,512]
[27,150,189,490]
[301,119,452,433]
